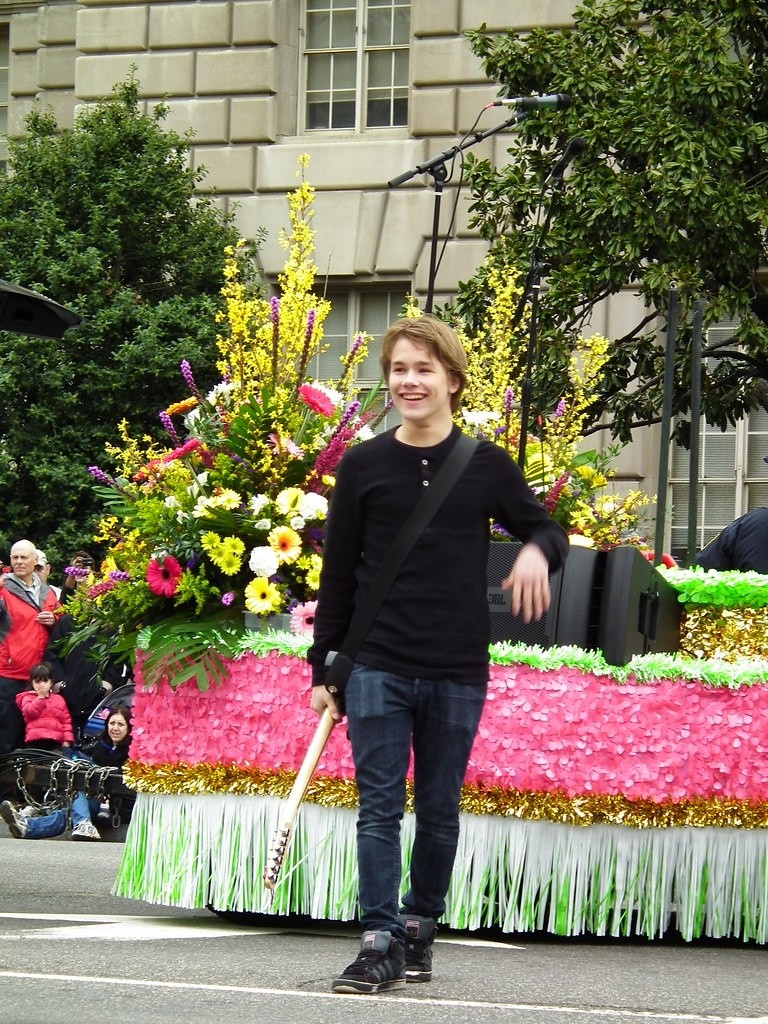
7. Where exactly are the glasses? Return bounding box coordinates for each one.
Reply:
[35,566,48,571]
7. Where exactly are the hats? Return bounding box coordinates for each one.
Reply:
[34,548,47,568]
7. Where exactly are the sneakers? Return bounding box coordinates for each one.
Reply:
[72,822,101,841]
[1,801,29,839]
[396,906,439,983]
[331,921,408,995]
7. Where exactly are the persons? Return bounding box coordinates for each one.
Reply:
[0,539,132,838]
[307,315,571,992]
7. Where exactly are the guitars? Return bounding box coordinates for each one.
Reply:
[263,639,346,890]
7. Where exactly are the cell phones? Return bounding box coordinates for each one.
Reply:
[78,558,93,566]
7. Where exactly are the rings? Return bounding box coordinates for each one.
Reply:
[46,619,48,622]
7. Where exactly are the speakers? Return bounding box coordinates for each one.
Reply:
[488,540,684,665]
